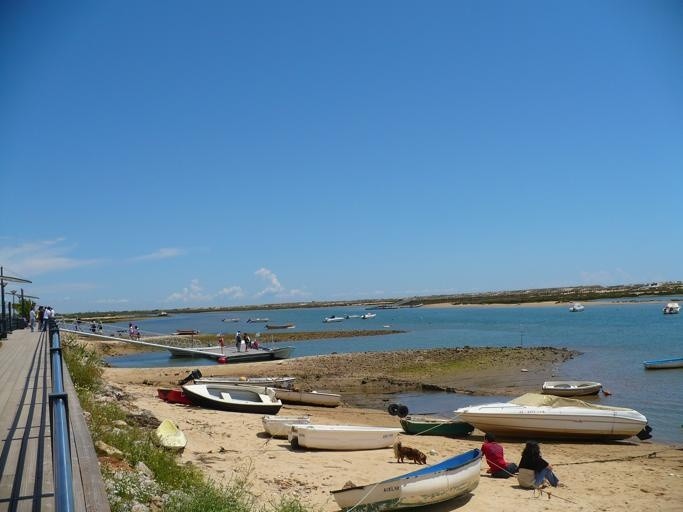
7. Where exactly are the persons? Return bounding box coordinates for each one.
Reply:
[218,335,224,353]
[235,330,258,353]
[518,441,564,489]
[30,306,54,332]
[75,317,103,335]
[128,323,140,339]
[482,431,518,479]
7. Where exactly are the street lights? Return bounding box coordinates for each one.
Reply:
[0,281,23,342]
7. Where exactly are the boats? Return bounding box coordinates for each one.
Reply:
[662,302,681,315]
[259,414,322,440]
[283,422,405,453]
[170,327,203,337]
[265,342,298,362]
[155,368,348,418]
[454,391,652,451]
[153,415,190,456]
[167,346,210,359]
[397,413,478,441]
[319,311,381,325]
[223,313,299,332]
[329,447,489,512]
[567,303,587,314]
[641,356,683,373]
[158,311,170,318]
[541,378,604,400]
[113,326,142,335]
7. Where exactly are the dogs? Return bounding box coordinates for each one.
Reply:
[394,439,426,466]
